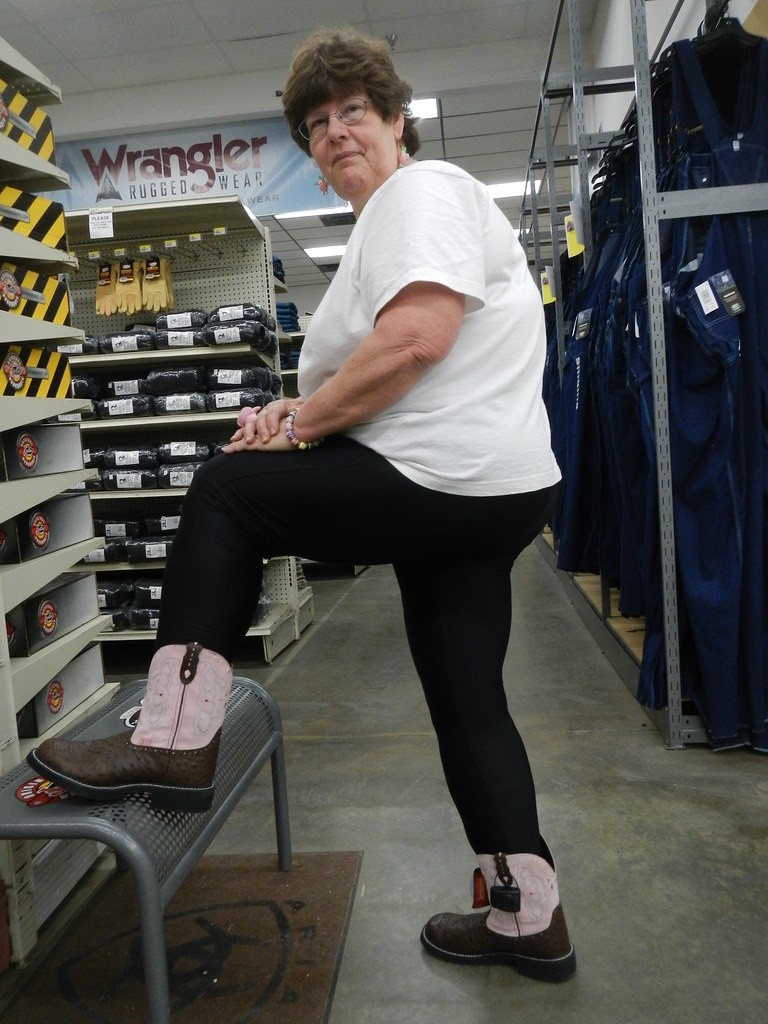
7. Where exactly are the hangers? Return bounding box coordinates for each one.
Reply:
[592,38,691,190]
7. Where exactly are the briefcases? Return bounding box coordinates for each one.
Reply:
[27,641,232,813]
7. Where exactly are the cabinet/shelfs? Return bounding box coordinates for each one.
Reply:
[0,52,313,963]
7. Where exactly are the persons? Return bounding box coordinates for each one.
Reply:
[24,29,579,983]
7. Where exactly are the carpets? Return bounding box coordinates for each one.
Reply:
[0,850,364,1024]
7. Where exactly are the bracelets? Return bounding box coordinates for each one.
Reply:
[285,408,324,450]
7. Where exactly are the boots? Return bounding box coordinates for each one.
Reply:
[422,836,577,984]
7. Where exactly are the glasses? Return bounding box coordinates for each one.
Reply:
[294,96,372,141]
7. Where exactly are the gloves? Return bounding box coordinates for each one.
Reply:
[96,259,174,317]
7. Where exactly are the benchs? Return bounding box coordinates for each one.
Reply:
[0,676,293,1024]
